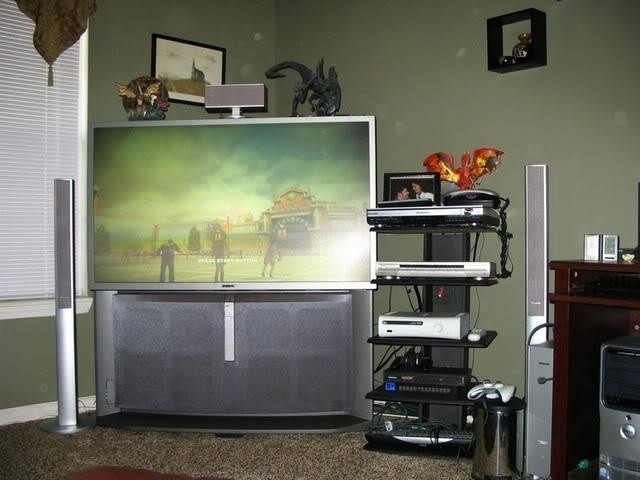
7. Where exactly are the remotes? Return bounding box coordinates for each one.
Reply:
[468,327,487,341]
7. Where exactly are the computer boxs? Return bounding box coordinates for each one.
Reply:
[595,336,640,480]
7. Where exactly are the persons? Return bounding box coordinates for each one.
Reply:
[412,180,435,202]
[396,188,411,199]
[158,227,289,282]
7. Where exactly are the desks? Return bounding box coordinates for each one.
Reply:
[546,257,640,479]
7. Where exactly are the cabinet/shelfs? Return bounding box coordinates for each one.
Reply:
[365,224,503,458]
[94,287,375,440]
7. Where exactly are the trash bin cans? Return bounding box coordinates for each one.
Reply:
[470,396,526,480]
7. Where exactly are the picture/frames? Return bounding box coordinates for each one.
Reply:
[149,33,227,105]
[383,171,441,205]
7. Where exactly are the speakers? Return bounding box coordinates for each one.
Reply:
[584,233,619,262]
[523,162,549,341]
[202,83,267,109]
[37,177,95,434]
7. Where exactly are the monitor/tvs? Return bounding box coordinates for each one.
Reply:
[85,116,375,291]
